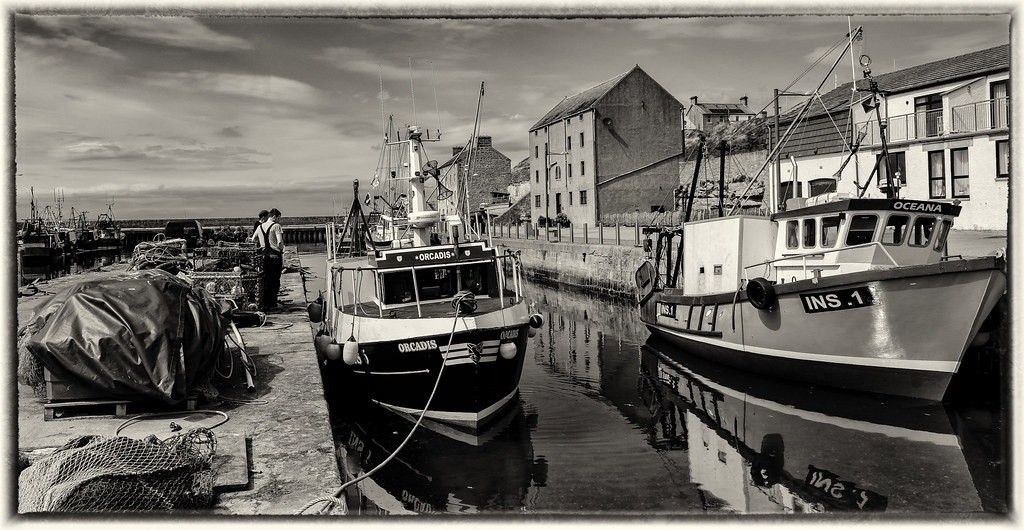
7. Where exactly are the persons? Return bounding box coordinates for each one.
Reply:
[251,208,285,313]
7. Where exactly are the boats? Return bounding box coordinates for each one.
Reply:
[304,53,545,456]
[631,13,1006,420]
[16,184,121,285]
[363,226,393,247]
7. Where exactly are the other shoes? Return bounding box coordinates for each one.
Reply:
[264,308,281,314]
